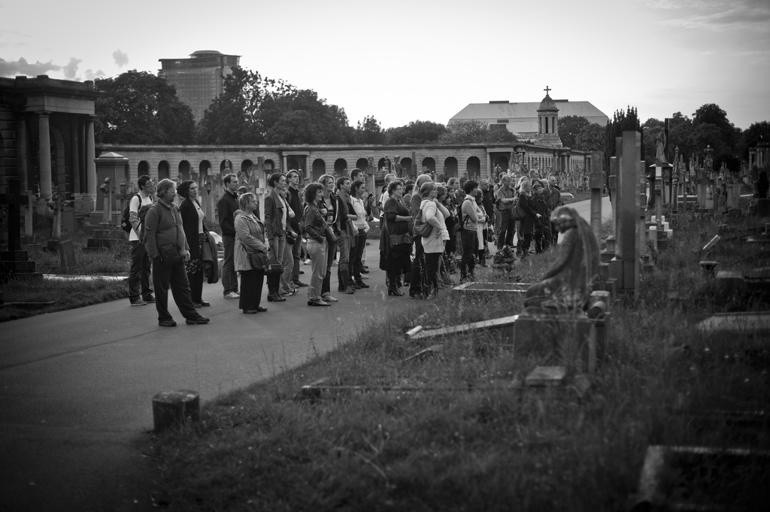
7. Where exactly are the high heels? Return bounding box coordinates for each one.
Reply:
[388,285,405,296]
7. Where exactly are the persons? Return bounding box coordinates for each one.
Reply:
[523,205,601,316]
[127,168,566,328]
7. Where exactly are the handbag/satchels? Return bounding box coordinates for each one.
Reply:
[248,251,283,276]
[121,192,152,232]
[139,204,162,241]
[326,227,337,244]
[413,209,433,237]
[286,224,297,244]
[162,245,184,268]
[389,232,411,250]
[512,204,524,220]
[483,222,495,241]
[456,198,473,231]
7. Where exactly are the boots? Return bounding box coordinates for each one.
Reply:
[267,273,285,302]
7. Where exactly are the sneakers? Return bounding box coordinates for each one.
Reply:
[159,318,176,326]
[192,300,209,308]
[131,298,147,305]
[186,314,209,324]
[279,255,370,306]
[244,304,266,313]
[143,294,156,303]
[224,290,240,298]
